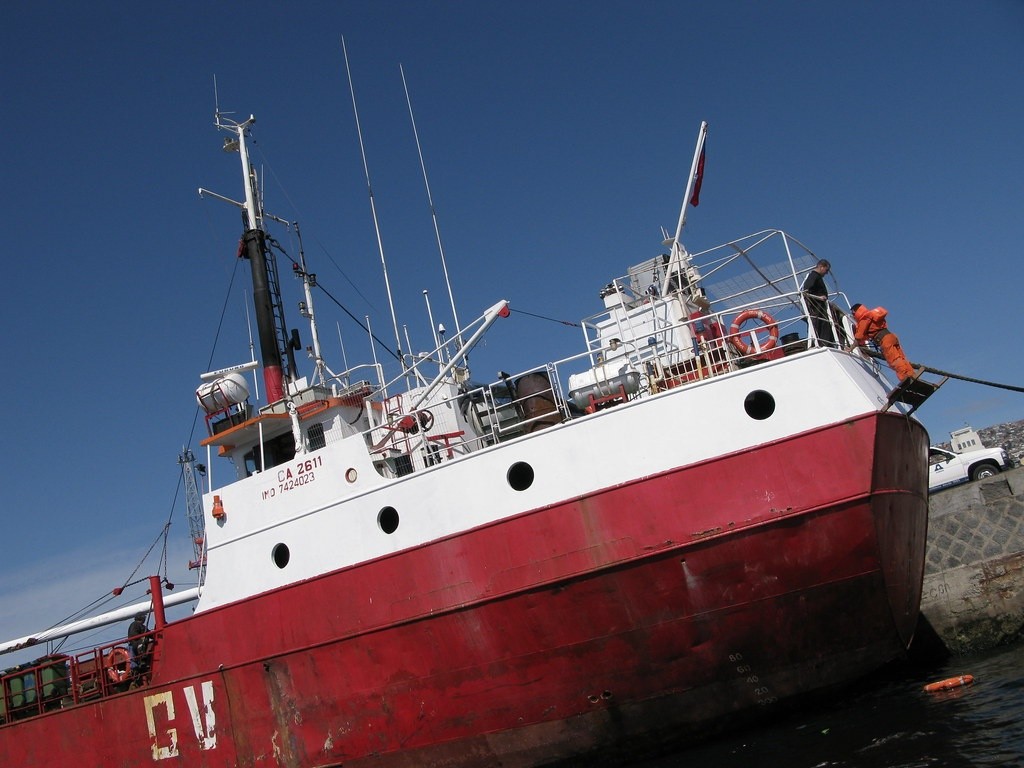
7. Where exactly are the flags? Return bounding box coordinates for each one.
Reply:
[690,138,705,207]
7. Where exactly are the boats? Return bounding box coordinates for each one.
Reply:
[1,35,948,768]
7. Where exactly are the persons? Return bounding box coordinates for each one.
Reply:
[128,612,154,689]
[800,259,839,347]
[843,303,915,381]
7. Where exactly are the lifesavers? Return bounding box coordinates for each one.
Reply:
[106,648,132,684]
[924,674,974,692]
[730,308,779,356]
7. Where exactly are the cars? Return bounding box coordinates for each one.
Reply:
[929,447,1009,494]
